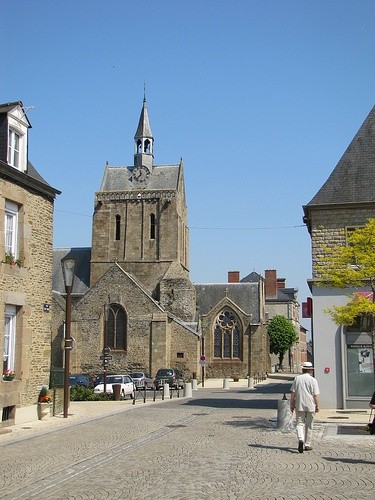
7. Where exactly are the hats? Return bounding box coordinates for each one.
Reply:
[301,361,314,369]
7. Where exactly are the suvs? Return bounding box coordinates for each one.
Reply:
[154,368,185,390]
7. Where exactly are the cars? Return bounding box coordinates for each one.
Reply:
[129,372,154,391]
[69,372,95,390]
[93,373,118,388]
[94,374,136,400]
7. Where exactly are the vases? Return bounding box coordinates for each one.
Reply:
[37,401,51,421]
[6,376,14,381]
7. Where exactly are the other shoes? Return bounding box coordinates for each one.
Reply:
[298,440,303,453]
[305,446,312,450]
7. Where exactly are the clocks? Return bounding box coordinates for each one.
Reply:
[132,165,150,183]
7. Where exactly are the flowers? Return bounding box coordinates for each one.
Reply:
[37,387,51,403]
[3,369,16,378]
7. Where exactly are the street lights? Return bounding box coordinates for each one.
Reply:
[60,250,79,418]
[199,313,208,387]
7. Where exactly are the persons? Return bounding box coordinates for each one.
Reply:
[290,361,320,453]
